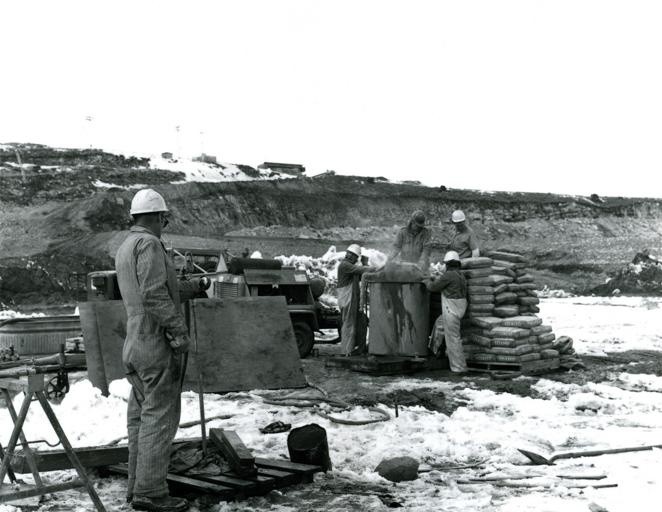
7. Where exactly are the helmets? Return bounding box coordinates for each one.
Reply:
[346,243,362,257]
[129,187,170,215]
[451,210,466,223]
[442,250,459,262]
[411,210,426,227]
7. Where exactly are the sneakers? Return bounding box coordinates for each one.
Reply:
[125,495,190,512]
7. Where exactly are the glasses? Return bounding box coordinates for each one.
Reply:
[159,213,169,228]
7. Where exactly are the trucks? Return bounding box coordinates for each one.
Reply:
[87,247,342,359]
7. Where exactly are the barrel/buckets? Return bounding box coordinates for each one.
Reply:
[369,278,430,357]
[288,423,332,471]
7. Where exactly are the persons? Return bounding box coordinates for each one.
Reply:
[446,210,481,260]
[421,251,471,377]
[377,209,433,273]
[114,187,213,511]
[334,243,375,357]
